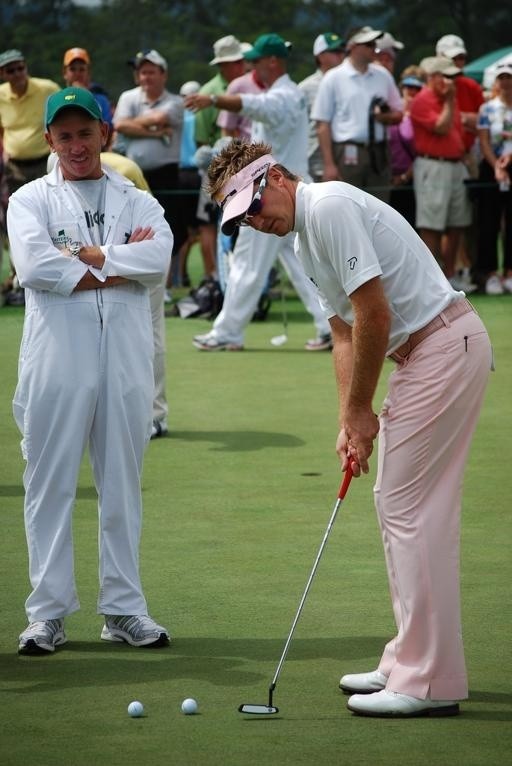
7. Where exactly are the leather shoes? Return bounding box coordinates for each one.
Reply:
[347,689,459,717]
[338,669,388,693]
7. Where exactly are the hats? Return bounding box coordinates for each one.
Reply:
[313,32,347,56]
[347,26,383,49]
[0,49,24,66]
[494,62,512,80]
[243,34,288,60]
[127,48,168,72]
[45,87,102,133]
[213,153,278,236]
[209,35,252,65]
[436,34,467,59]
[375,32,404,50]
[403,75,426,87]
[180,81,201,96]
[425,57,461,76]
[64,47,90,66]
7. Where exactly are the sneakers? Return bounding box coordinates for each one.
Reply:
[450,268,512,295]
[193,334,244,351]
[151,421,167,437]
[305,333,333,351]
[18,615,67,653]
[100,614,171,648]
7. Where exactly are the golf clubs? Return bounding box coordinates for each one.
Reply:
[271,260,288,346]
[239,456,355,714]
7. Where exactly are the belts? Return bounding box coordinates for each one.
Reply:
[417,153,458,163]
[387,298,473,363]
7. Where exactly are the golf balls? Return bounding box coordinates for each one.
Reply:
[182,698,197,714]
[128,701,143,716]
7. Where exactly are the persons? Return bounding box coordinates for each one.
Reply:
[46,92,169,440]
[4,86,174,656]
[201,135,493,718]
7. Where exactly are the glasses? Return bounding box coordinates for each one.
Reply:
[234,163,270,226]
[6,65,24,74]
